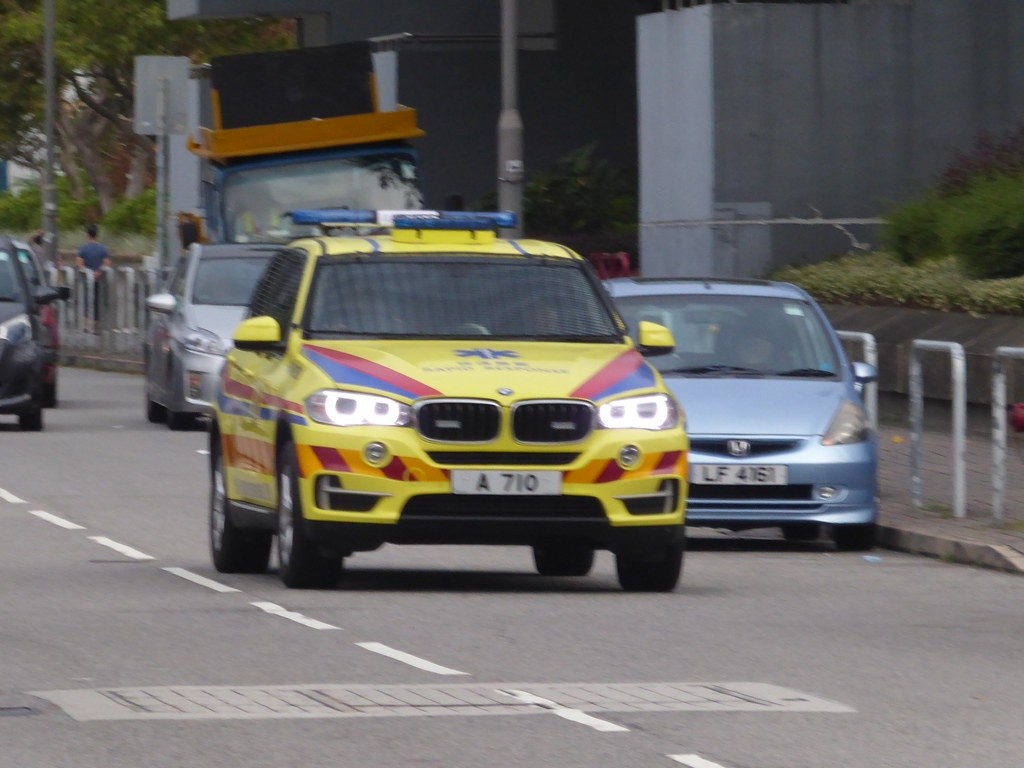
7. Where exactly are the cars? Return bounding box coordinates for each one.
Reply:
[0,236,78,437]
[595,272,882,541]
[149,245,293,432]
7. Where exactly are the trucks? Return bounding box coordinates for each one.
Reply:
[205,146,435,282]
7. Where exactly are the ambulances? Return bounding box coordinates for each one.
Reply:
[206,209,695,594]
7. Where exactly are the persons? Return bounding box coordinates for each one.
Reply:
[29,223,111,335]
